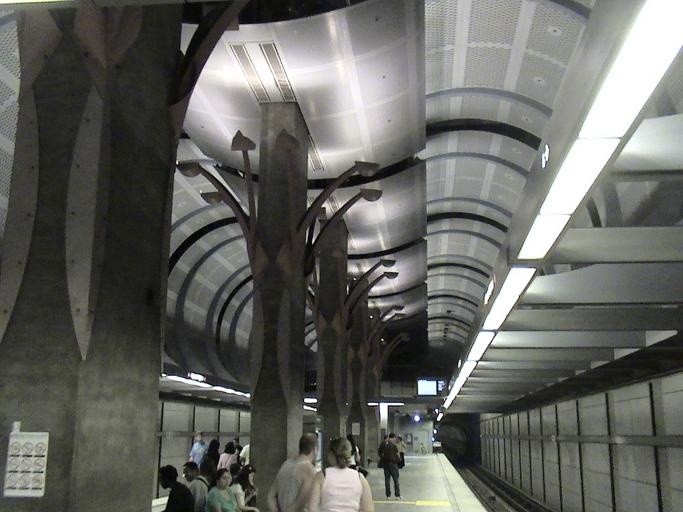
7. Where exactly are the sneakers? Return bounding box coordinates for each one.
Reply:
[387,497,392,500]
[396,496,403,500]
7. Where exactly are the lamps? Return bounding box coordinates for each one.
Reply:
[436,1,683,421]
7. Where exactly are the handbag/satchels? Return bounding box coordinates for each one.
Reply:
[377,457,385,468]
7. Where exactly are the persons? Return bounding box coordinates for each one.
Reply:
[267,432,374,512]
[381,434,407,468]
[378,432,403,500]
[158,430,260,511]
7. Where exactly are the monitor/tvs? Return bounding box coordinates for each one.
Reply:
[416,377,438,397]
